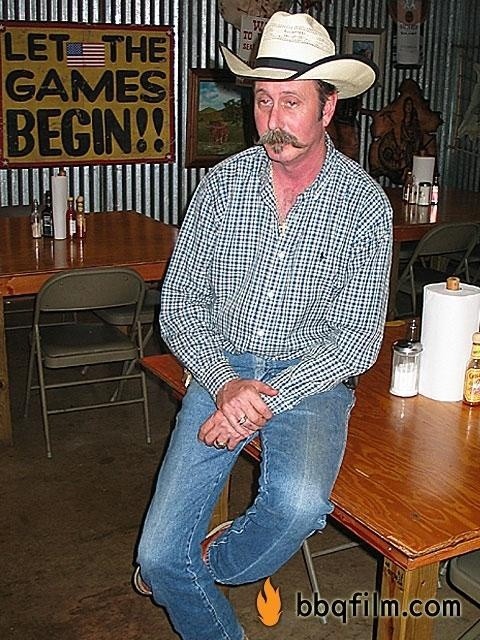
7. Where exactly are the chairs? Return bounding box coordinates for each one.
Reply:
[446,550,480,640]
[22,267,151,459]
[81,281,161,402]
[396,221,480,318]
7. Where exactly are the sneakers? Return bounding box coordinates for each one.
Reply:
[132,519,235,598]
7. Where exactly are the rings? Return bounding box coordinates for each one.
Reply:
[216,437,228,450]
[237,414,249,425]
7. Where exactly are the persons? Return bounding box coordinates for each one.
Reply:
[399,95,424,167]
[128,11,394,637]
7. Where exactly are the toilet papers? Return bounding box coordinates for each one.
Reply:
[53,238,68,268]
[417,282,480,404]
[412,154,435,205]
[51,175,69,240]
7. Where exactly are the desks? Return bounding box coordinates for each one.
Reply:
[136,317,480,640]
[0,210,179,447]
[381,185,480,322]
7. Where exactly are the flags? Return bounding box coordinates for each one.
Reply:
[66,42,106,67]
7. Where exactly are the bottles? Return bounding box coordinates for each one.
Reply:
[388,401,420,430]
[402,170,440,207]
[32,238,54,269]
[390,320,422,398]
[29,191,55,237]
[402,204,439,224]
[463,331,480,405]
[459,404,480,438]
[66,195,87,240]
[66,240,86,267]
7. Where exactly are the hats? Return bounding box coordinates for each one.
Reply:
[217,10,380,101]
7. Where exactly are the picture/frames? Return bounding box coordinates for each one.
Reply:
[341,26,385,88]
[185,66,257,169]
